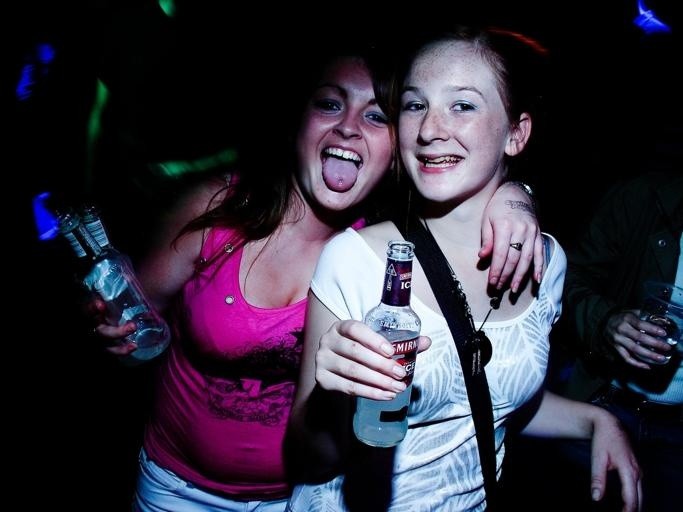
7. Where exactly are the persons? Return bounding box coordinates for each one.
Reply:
[284,21,644,512]
[561,172,683,512]
[79,47,544,512]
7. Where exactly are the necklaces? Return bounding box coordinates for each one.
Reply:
[424,218,504,377]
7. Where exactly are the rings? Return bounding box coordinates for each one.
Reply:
[511,242,522,250]
[87,328,98,338]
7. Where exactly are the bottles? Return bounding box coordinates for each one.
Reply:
[41,168,172,361]
[352,241,420,452]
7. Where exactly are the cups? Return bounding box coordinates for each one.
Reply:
[633,281,682,396]
